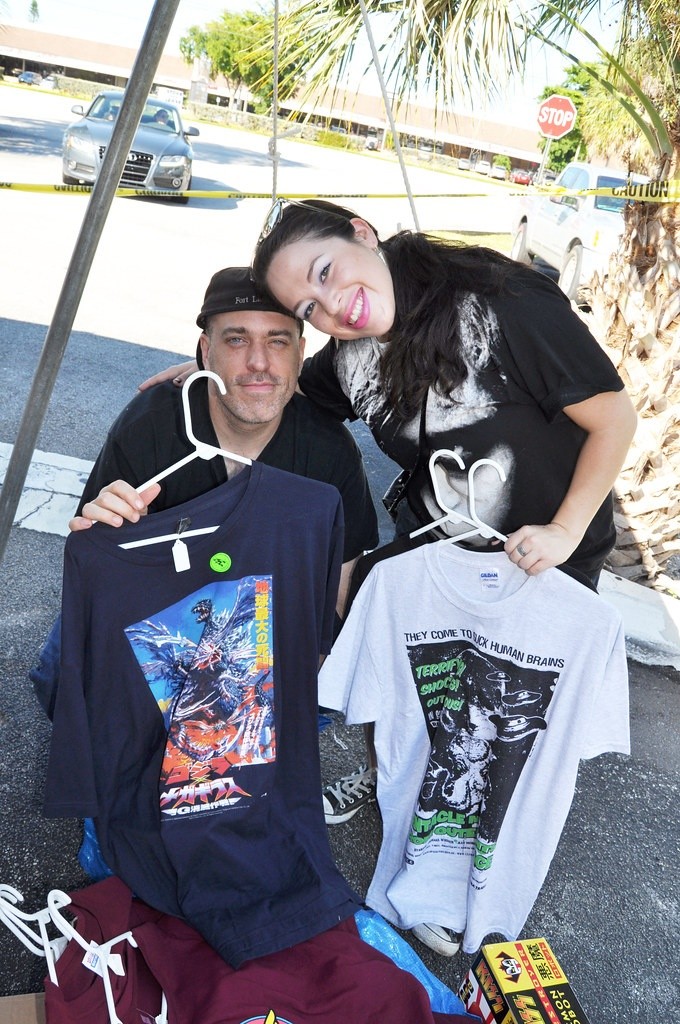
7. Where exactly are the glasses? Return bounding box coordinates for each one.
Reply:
[251,196,351,282]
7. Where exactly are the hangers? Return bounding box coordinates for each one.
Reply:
[451,459,509,548]
[87,368,255,549]
[0,883,74,988]
[408,448,481,539]
[46,887,170,1024]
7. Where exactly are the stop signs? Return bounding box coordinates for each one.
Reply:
[535,95,579,144]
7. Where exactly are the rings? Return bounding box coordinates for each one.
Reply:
[516,543,527,557]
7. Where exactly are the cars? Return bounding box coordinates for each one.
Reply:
[42,72,58,88]
[491,165,508,182]
[458,157,471,170]
[513,171,531,186]
[59,91,200,204]
[18,71,42,86]
[419,145,434,162]
[5,66,22,77]
[477,160,491,175]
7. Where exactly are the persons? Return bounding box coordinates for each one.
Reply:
[105,111,116,121]
[32,267,368,727]
[135,200,638,825]
[153,109,169,125]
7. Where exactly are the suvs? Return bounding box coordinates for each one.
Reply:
[507,163,655,316]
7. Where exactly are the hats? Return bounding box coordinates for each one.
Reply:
[196,267,304,371]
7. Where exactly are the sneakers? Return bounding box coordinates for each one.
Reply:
[322,764,378,825]
[413,921,461,957]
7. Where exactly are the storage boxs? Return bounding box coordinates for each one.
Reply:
[455,937,591,1024]
